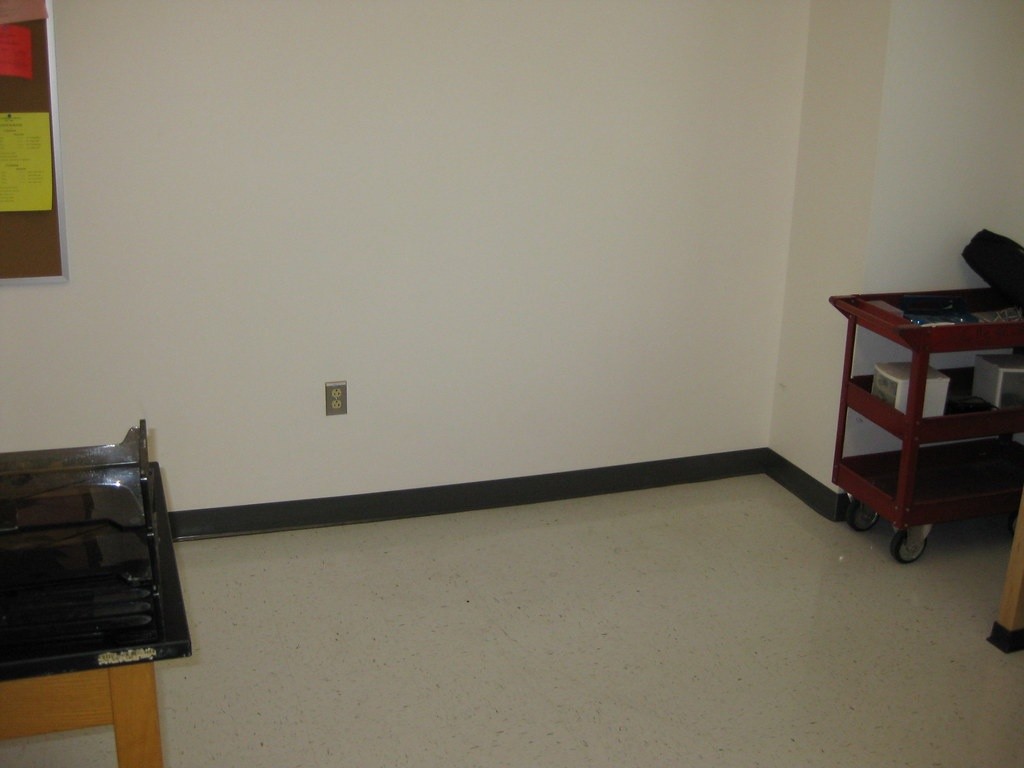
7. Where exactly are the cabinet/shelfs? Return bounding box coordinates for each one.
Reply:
[830,286,1024,527]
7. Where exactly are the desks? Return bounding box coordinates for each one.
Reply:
[0,460,196,768]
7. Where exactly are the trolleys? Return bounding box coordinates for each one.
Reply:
[829,285,1024,564]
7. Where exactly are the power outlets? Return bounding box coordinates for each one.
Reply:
[327,382,350,414]
